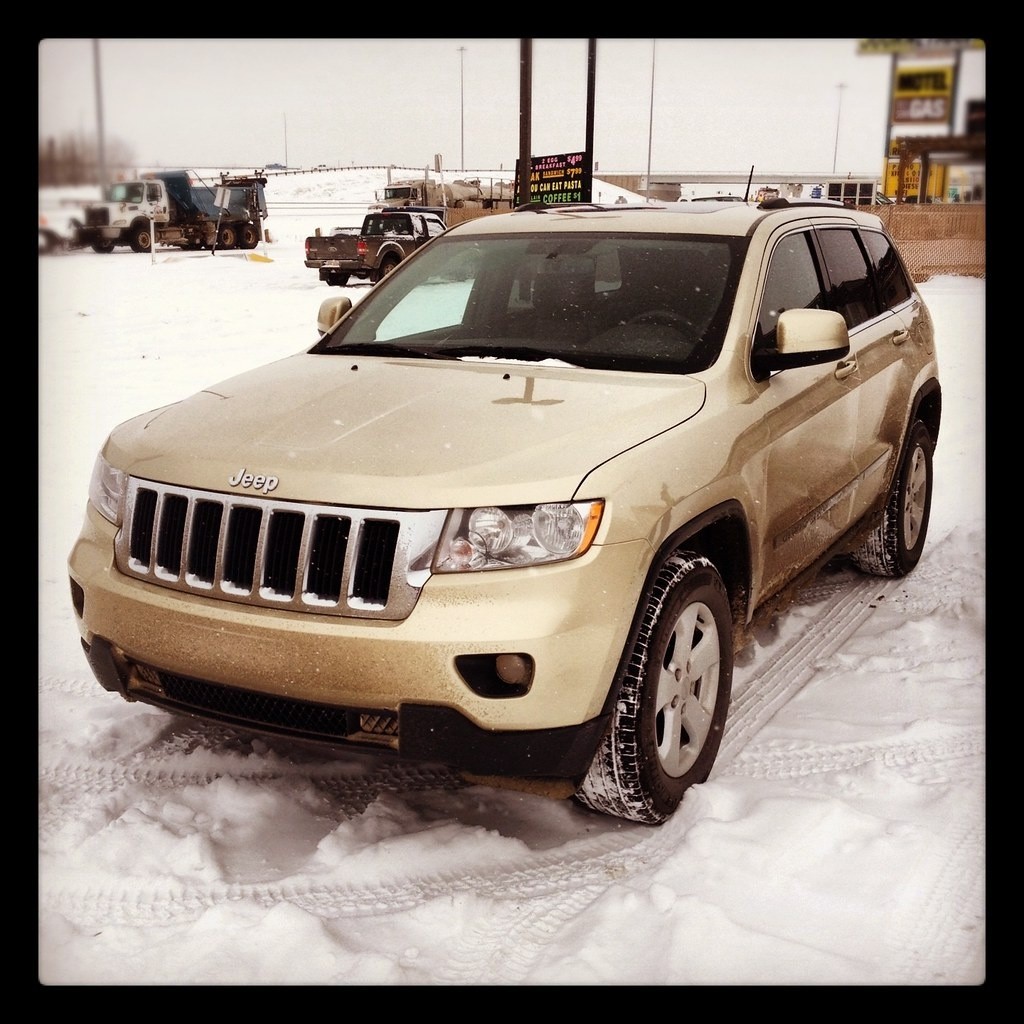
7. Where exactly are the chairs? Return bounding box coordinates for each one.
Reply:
[501,271,595,342]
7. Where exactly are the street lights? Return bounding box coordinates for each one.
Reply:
[458,45,468,172]
[833,82,848,173]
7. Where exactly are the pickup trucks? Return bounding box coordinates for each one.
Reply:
[330,206,448,237]
[303,210,481,291]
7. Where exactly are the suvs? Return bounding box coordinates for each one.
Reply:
[67,199,943,823]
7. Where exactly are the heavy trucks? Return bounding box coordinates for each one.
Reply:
[368,176,515,215]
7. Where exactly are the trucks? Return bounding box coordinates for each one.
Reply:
[74,169,267,253]
[38,185,102,255]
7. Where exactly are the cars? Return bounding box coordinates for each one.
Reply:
[876,192,896,205]
